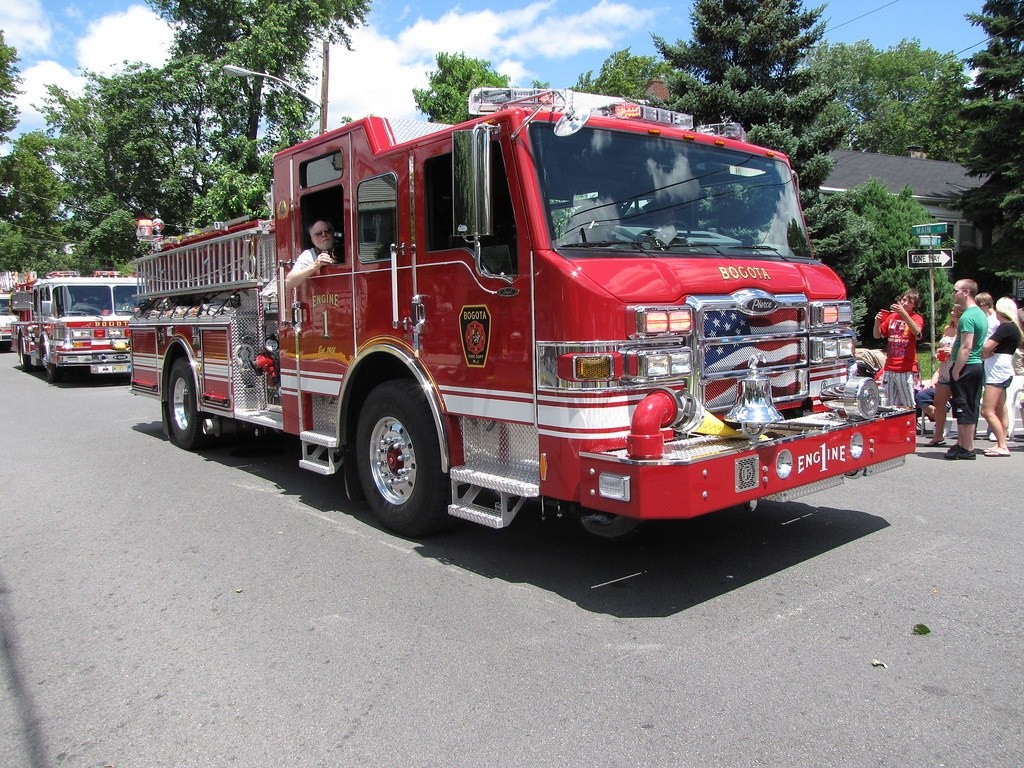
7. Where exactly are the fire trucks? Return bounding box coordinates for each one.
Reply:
[10,268,153,383]
[0,270,42,350]
[123,84,920,540]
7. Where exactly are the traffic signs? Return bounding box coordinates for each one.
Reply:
[912,222,948,235]
[907,248,954,269]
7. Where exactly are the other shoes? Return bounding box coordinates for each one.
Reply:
[945,445,976,459]
[987,431,997,442]
[933,425,937,434]
[942,421,952,438]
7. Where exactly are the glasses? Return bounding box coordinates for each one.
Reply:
[953,289,966,293]
[311,227,333,238]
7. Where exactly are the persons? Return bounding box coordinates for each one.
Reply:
[913,361,952,437]
[973,293,999,439]
[874,290,924,408]
[925,304,964,448]
[286,218,344,287]
[855,349,886,381]
[945,279,988,460]
[981,297,1024,456]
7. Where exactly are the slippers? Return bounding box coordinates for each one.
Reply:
[982,447,1011,457]
[924,441,947,447]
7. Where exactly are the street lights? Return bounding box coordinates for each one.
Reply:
[224,41,332,137]
